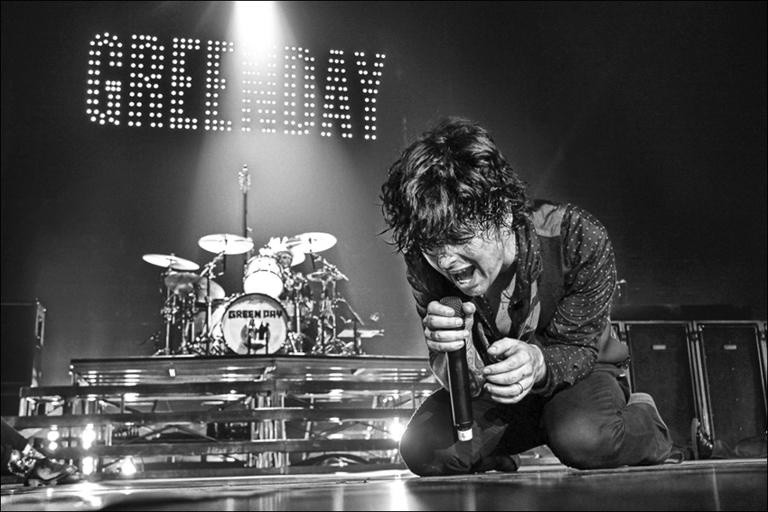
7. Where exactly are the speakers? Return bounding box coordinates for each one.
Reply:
[610,318,768,460]
[0,299,48,388]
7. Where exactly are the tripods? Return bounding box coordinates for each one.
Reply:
[148,265,366,356]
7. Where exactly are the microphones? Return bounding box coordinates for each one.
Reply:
[438,296,474,442]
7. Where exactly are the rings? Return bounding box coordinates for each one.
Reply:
[516,382,524,394]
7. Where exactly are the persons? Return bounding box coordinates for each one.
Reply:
[378,114,675,478]
[278,251,318,359]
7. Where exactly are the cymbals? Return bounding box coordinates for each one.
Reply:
[295,232,337,253]
[142,254,200,271]
[197,233,253,254]
[165,273,226,303]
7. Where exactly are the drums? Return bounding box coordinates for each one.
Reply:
[242,255,284,299]
[211,293,293,355]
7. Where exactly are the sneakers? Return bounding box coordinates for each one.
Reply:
[691,417,714,462]
[483,454,521,474]
[628,393,672,466]
